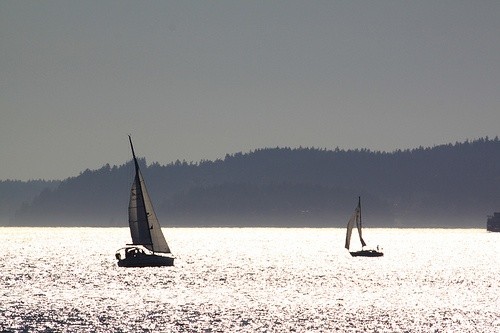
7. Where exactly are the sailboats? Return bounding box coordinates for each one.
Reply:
[116,135,176,266]
[345,195,383,257]
